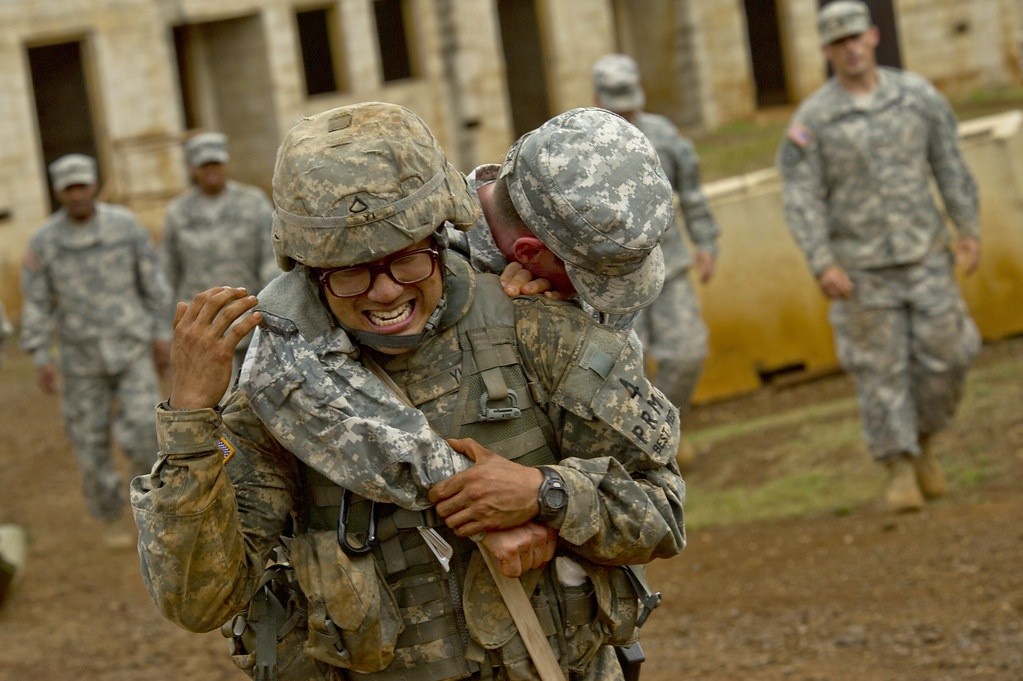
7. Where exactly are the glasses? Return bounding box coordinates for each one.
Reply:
[318,240,439,298]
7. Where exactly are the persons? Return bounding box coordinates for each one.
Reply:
[127,102,686,680]
[776,2,985,514]
[153,134,285,400]
[19,151,169,552]
[231,108,677,578]
[592,54,721,420]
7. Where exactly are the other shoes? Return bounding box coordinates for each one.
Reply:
[916,433,947,497]
[101,519,135,550]
[881,452,925,515]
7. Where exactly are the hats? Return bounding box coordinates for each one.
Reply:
[815,1,871,44]
[50,154,97,191]
[594,53,645,113]
[185,132,229,168]
[501,108,673,313]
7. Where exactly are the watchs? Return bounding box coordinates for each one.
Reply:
[533,465,567,523]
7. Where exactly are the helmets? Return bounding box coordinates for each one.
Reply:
[270,100,478,274]
